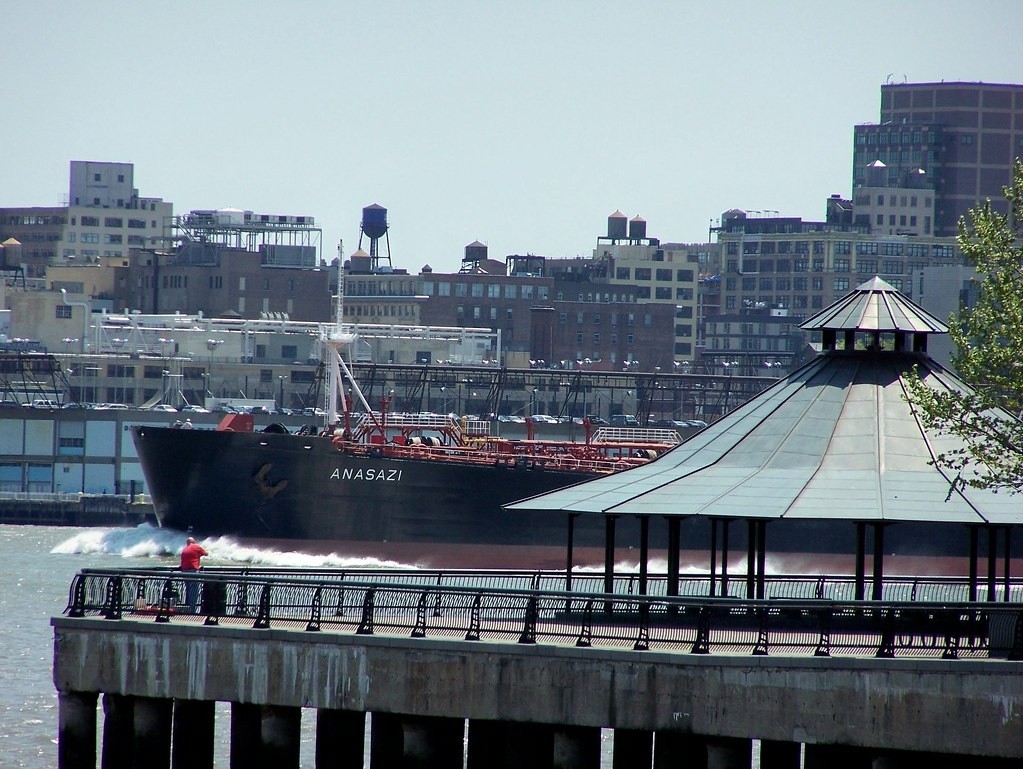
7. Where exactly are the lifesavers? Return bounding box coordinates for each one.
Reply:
[332,437,345,453]
[615,460,629,471]
[561,454,576,472]
[532,459,545,472]
[419,444,430,459]
[408,444,418,459]
[495,457,509,470]
[514,457,527,472]
[370,447,384,460]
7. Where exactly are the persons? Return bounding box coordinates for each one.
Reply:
[174,418,192,429]
[181,537,208,614]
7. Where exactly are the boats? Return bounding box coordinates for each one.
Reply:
[128,238,1023,613]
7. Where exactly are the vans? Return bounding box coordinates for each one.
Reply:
[302,407,327,417]
[610,414,638,427]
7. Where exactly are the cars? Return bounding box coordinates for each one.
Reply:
[533,414,558,424]
[211,405,237,415]
[507,416,526,424]
[276,408,293,416]
[252,407,278,416]
[674,420,689,427]
[153,404,177,413]
[182,404,210,414]
[0,399,128,414]
